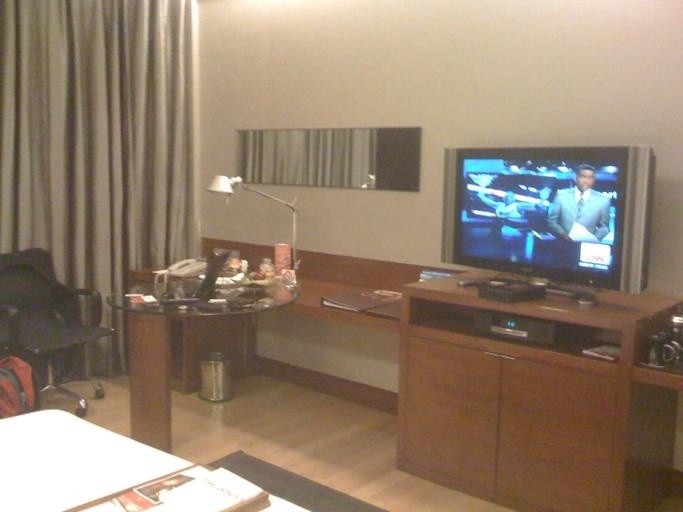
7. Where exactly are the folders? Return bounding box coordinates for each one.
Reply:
[321,293,389,313]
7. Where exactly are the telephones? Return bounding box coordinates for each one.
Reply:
[167,259,208,277]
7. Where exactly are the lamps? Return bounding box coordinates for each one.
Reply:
[206,172,300,282]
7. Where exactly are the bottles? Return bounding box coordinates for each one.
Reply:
[259,257,275,282]
[669,305,683,359]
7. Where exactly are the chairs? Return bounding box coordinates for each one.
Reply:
[0,247,116,417]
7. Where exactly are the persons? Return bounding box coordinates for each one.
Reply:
[476,187,552,261]
[546,164,611,242]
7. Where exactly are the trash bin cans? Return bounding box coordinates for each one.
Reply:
[198,352,234,403]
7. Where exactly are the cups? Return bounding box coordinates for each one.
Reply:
[644,329,681,368]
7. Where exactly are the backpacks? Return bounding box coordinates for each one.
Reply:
[0,356,41,420]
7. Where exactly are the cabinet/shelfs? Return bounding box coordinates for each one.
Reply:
[395,267,683,512]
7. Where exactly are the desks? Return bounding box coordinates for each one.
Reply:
[105,277,301,404]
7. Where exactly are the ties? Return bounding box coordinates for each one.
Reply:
[576,192,585,216]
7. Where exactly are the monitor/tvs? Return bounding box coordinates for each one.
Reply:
[438,145,659,307]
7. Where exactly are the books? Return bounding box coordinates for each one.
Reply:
[130,294,160,309]
[582,343,621,362]
[77,466,269,512]
[321,287,403,312]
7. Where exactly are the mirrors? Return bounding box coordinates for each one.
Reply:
[236,127,422,193]
